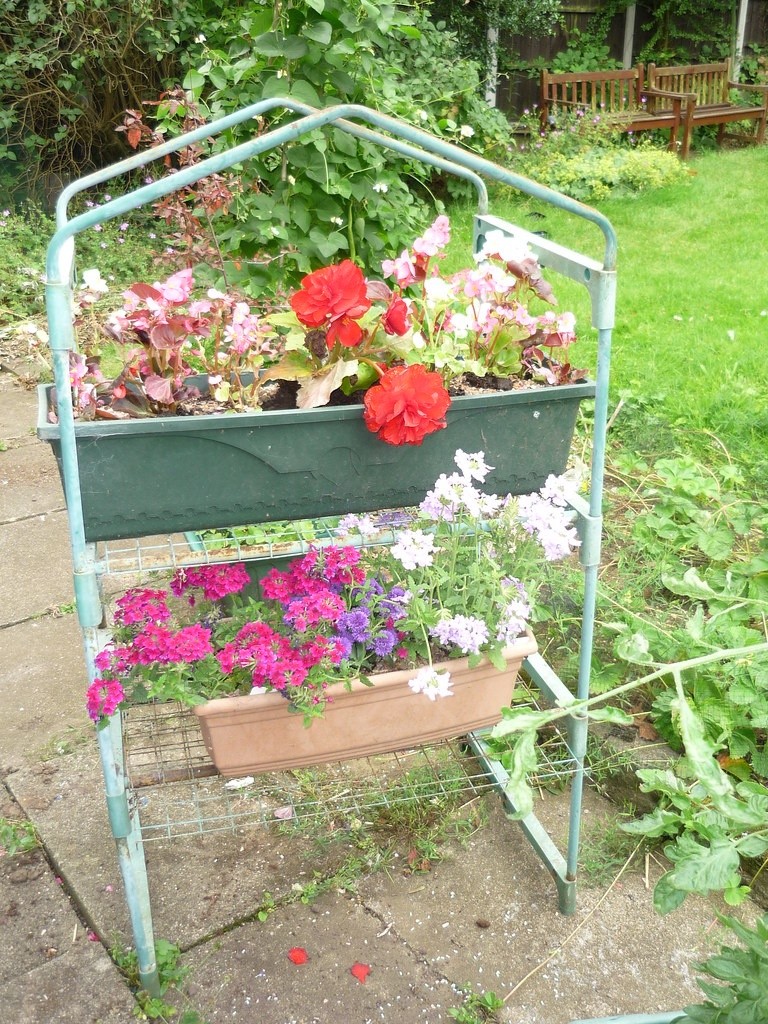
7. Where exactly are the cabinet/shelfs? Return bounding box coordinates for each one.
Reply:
[42,95,621,993]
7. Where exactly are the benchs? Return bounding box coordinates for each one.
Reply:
[540,58,768,161]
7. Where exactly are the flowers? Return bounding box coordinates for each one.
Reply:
[24,215,591,448]
[85,443,587,730]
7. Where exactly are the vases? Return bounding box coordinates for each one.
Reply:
[194,626,538,779]
[34,353,596,542]
